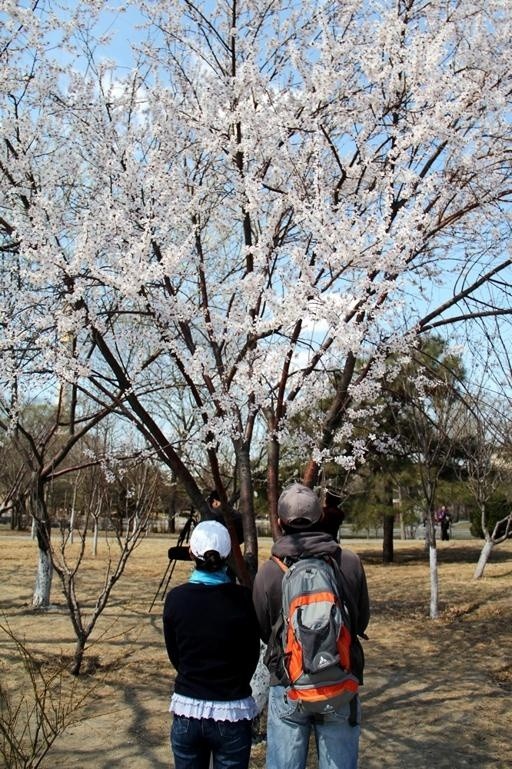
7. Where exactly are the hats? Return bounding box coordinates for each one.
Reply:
[207,489,227,503]
[278,483,322,528]
[190,518,233,563]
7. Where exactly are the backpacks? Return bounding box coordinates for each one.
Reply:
[263,553,365,714]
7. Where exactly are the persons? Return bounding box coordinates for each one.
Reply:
[250,483,371,769]
[437,504,452,541]
[199,489,244,585]
[161,519,260,768]
